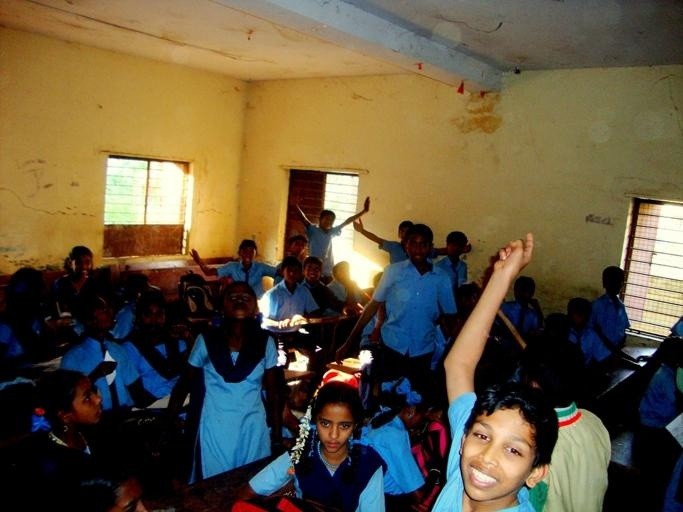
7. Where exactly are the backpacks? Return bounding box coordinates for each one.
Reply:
[177,269,218,323]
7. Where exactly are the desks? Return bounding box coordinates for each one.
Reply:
[267,311,357,388]
[159,454,288,512]
[596,348,657,482]
[0,351,60,490]
